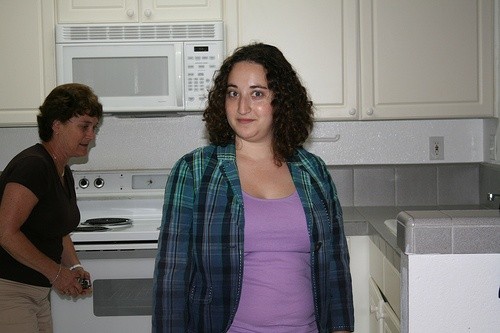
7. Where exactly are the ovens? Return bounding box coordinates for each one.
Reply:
[49,250,158,333]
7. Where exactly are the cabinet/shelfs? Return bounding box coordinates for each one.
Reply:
[0,0,57,129]
[222,0,494,122]
[368,240,401,333]
[57,0,223,23]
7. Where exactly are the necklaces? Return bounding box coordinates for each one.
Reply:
[45,142,65,176]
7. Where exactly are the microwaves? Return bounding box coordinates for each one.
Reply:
[55,40,228,112]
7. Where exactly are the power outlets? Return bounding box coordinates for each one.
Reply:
[428,136,444,161]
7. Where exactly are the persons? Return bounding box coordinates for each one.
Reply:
[0,84,103,333]
[148,42,355,333]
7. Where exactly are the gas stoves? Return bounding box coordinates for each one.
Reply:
[69,168,170,242]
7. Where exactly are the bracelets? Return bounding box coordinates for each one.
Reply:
[69,264,83,270]
[50,263,61,284]
